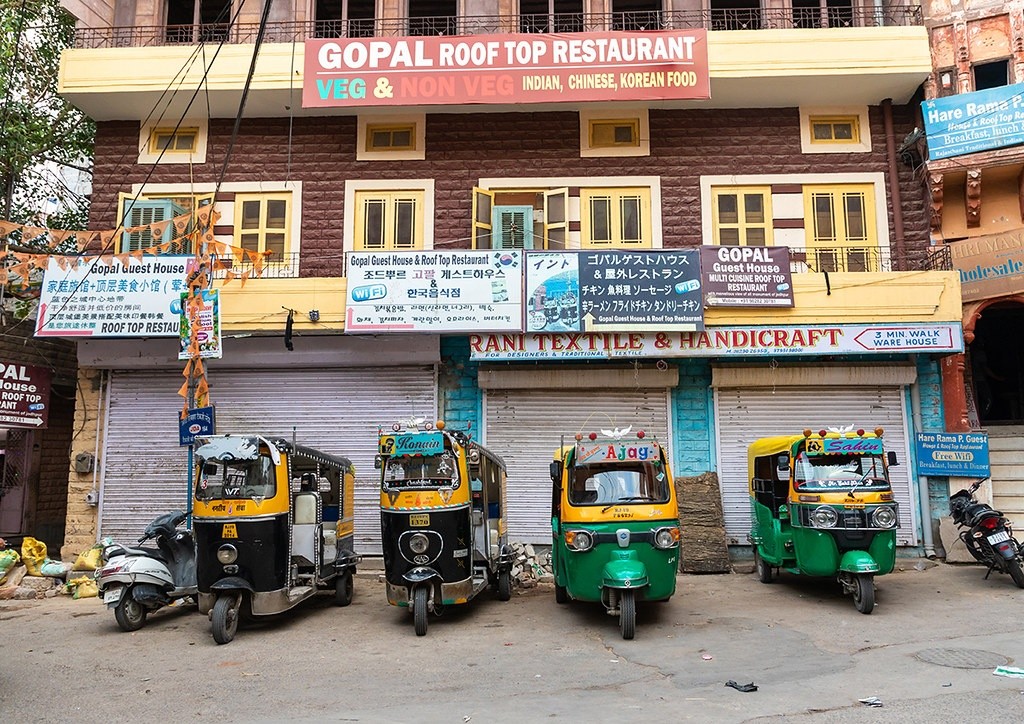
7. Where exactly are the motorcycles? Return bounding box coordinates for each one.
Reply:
[545,423,686,640]
[188,427,369,647]
[948,474,1024,588]
[368,418,516,637]
[745,422,905,615]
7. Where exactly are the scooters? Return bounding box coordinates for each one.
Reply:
[95,508,197,633]
